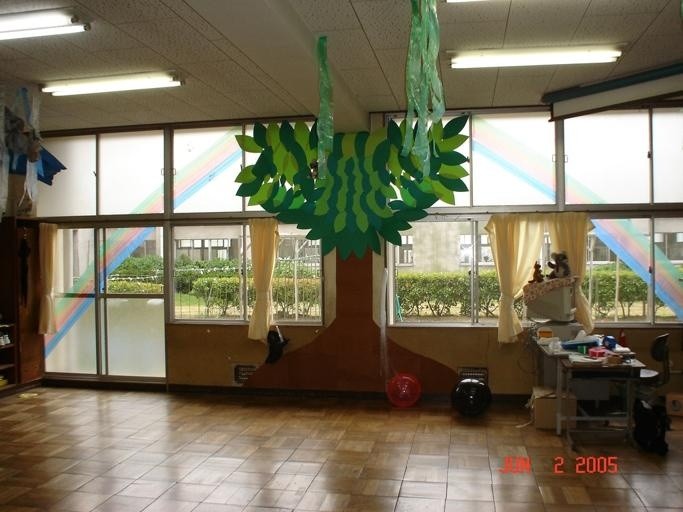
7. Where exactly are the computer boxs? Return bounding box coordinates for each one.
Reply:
[538,320,584,341]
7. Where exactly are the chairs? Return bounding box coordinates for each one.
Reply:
[602,329,672,428]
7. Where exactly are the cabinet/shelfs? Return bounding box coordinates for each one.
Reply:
[0,224,47,396]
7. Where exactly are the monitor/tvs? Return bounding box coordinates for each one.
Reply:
[526,279,578,324]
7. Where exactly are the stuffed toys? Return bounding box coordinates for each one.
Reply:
[546,249,571,279]
[527,261,545,284]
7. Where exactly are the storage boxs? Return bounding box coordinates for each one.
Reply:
[531,384,580,433]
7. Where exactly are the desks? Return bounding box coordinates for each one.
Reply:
[527,330,646,441]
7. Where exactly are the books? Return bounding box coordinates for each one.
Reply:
[568,354,604,366]
